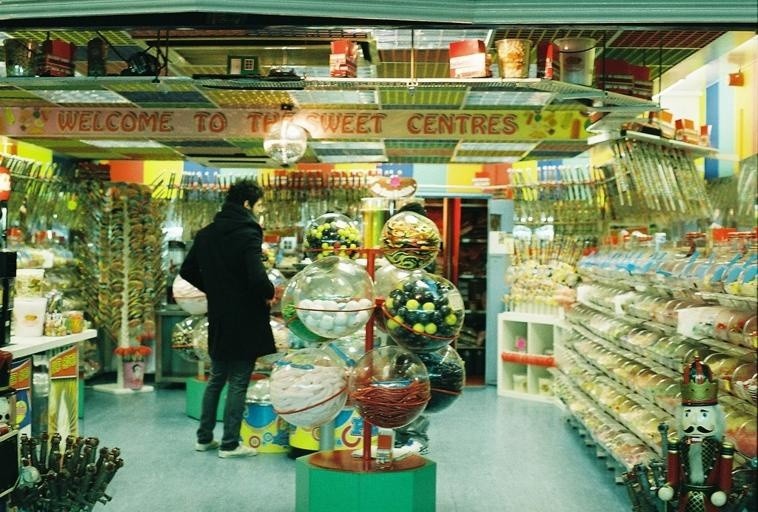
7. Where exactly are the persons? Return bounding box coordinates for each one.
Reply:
[658,358,734,512]
[179,177,275,458]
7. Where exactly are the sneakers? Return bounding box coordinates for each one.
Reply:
[219,443,257,459]
[195,437,221,452]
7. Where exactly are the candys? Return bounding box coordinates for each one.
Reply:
[87,181,168,332]
[303,222,363,262]
[385,275,463,347]
[281,298,371,343]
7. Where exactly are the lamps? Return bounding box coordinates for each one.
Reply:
[258,115,311,168]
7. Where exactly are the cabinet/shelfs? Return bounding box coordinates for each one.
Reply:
[553,266,758,486]
[493,309,563,403]
[151,305,208,388]
